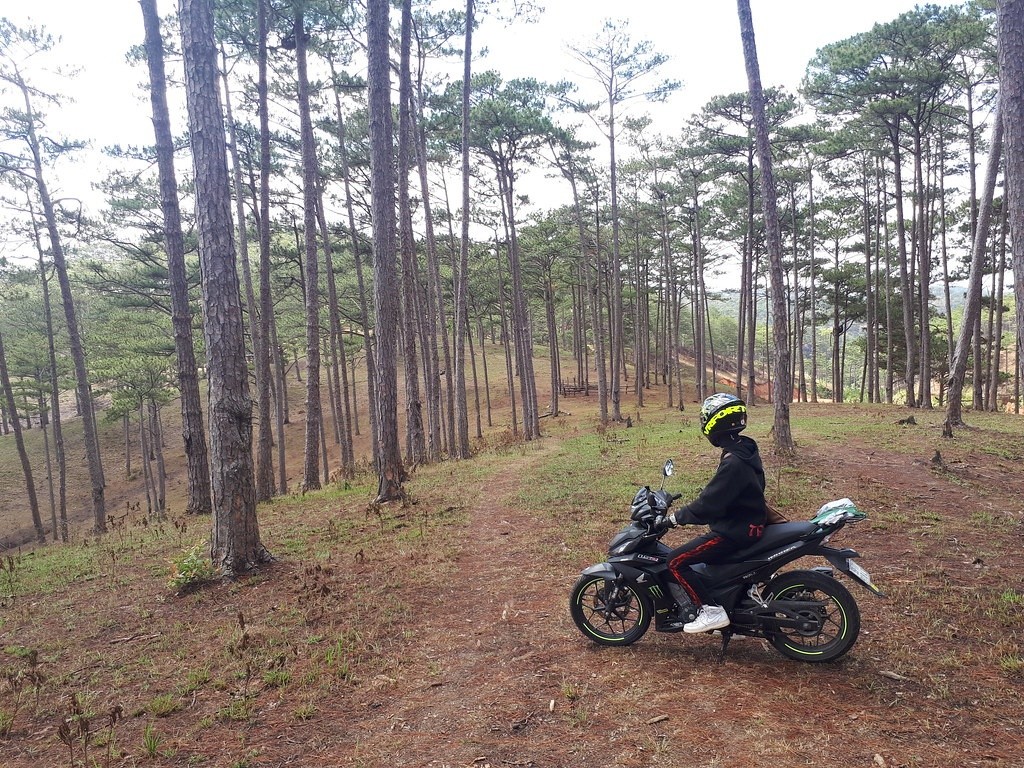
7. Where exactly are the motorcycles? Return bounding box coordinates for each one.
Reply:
[569,458,888,669]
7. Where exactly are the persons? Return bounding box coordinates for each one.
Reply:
[652,391,767,635]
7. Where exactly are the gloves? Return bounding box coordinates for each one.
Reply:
[652,512,677,533]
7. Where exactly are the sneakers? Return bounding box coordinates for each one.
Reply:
[713,629,745,640]
[683,604,731,632]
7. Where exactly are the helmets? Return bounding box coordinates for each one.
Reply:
[699,393,747,447]
